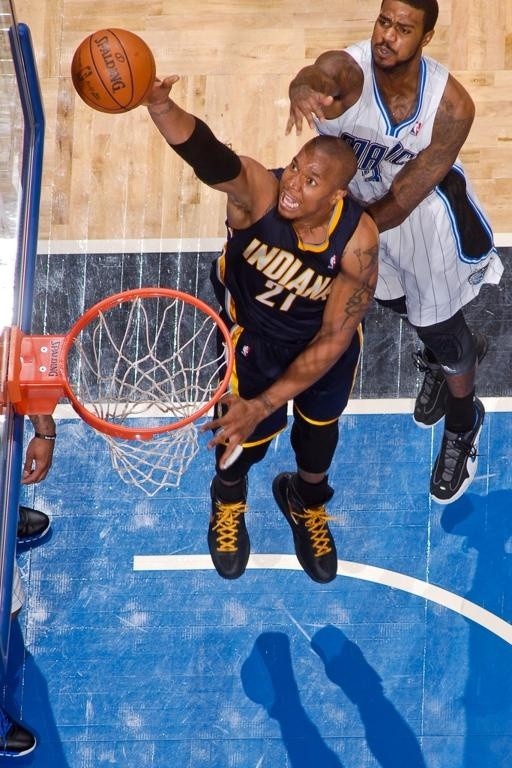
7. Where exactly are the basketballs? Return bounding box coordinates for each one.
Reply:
[70,28,155,110]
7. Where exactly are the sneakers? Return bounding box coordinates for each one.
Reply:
[16,506,51,544]
[0,705,37,758]
[412,332,489,505]
[207,474,250,580]
[272,471,337,584]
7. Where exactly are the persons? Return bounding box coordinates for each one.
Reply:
[1,412,57,758]
[140,74,380,582]
[285,0,504,505]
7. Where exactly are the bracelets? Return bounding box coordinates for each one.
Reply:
[34,432,59,440]
